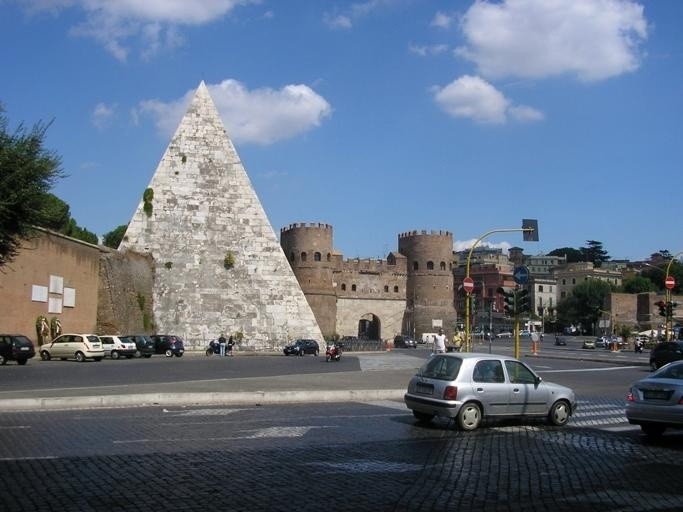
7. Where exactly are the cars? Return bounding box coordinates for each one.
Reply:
[467,327,544,342]
[564,327,579,335]
[555,336,567,346]
[624,358,682,437]
[403,351,577,432]
[636,337,650,344]
[584,335,623,350]
[649,340,682,373]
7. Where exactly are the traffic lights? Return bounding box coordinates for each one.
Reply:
[516,289,530,315]
[668,299,678,319]
[657,301,666,317]
[496,287,515,317]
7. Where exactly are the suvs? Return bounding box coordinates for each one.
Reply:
[0,333,35,365]
[395,334,418,349]
[284,339,320,355]
[38,331,184,362]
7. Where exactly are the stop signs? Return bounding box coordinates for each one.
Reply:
[664,276,676,289]
[462,277,475,294]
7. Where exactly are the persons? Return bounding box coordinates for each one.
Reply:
[432,328,448,354]
[635,339,641,350]
[426,336,429,347]
[226,335,234,356]
[333,342,339,358]
[219,334,226,357]
[452,326,464,352]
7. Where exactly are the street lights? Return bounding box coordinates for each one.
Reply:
[642,252,683,342]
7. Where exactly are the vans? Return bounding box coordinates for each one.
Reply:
[419,333,439,344]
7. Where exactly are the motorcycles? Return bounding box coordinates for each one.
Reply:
[206,337,235,358]
[634,343,643,353]
[326,344,343,361]
